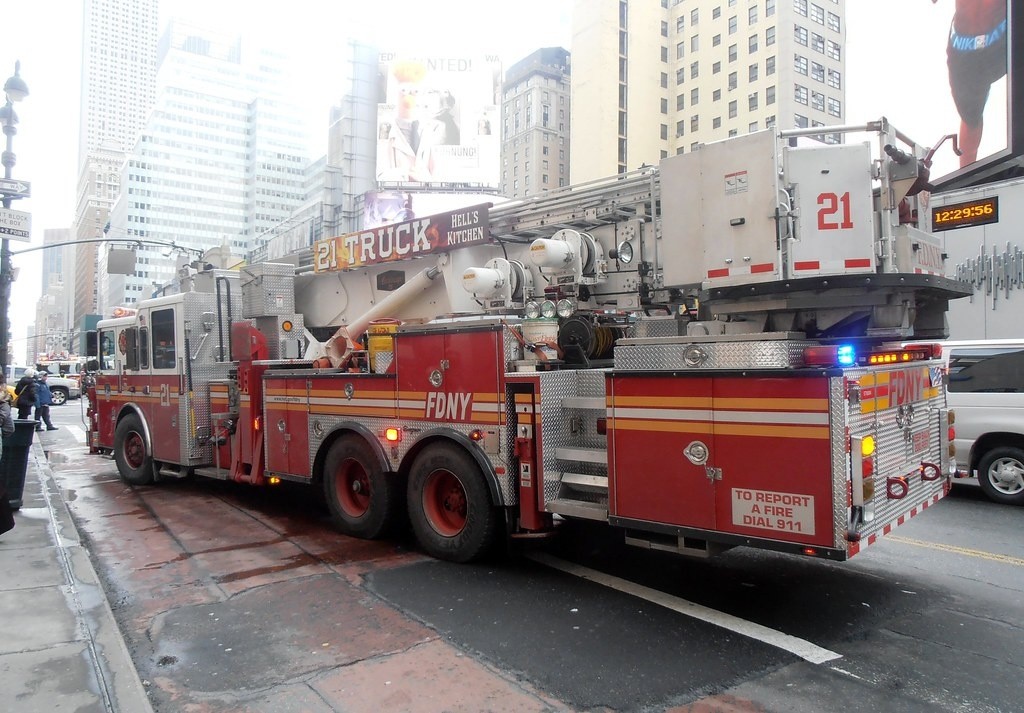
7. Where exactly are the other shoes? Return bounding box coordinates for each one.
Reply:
[35,428,45,431]
[47,426,59,429]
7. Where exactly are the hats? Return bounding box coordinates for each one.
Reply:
[38,370,48,380]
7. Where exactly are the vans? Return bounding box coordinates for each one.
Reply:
[911,338,1024,506]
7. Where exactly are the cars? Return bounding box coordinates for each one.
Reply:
[5,365,79,406]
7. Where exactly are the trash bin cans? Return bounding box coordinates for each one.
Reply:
[6,419,41,511]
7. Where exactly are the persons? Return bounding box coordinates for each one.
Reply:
[932,0,1008,167]
[14,369,57,431]
[0,374,15,535]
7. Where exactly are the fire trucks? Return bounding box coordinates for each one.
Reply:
[83,117,964,568]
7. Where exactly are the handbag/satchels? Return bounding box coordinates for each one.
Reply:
[8,392,19,405]
